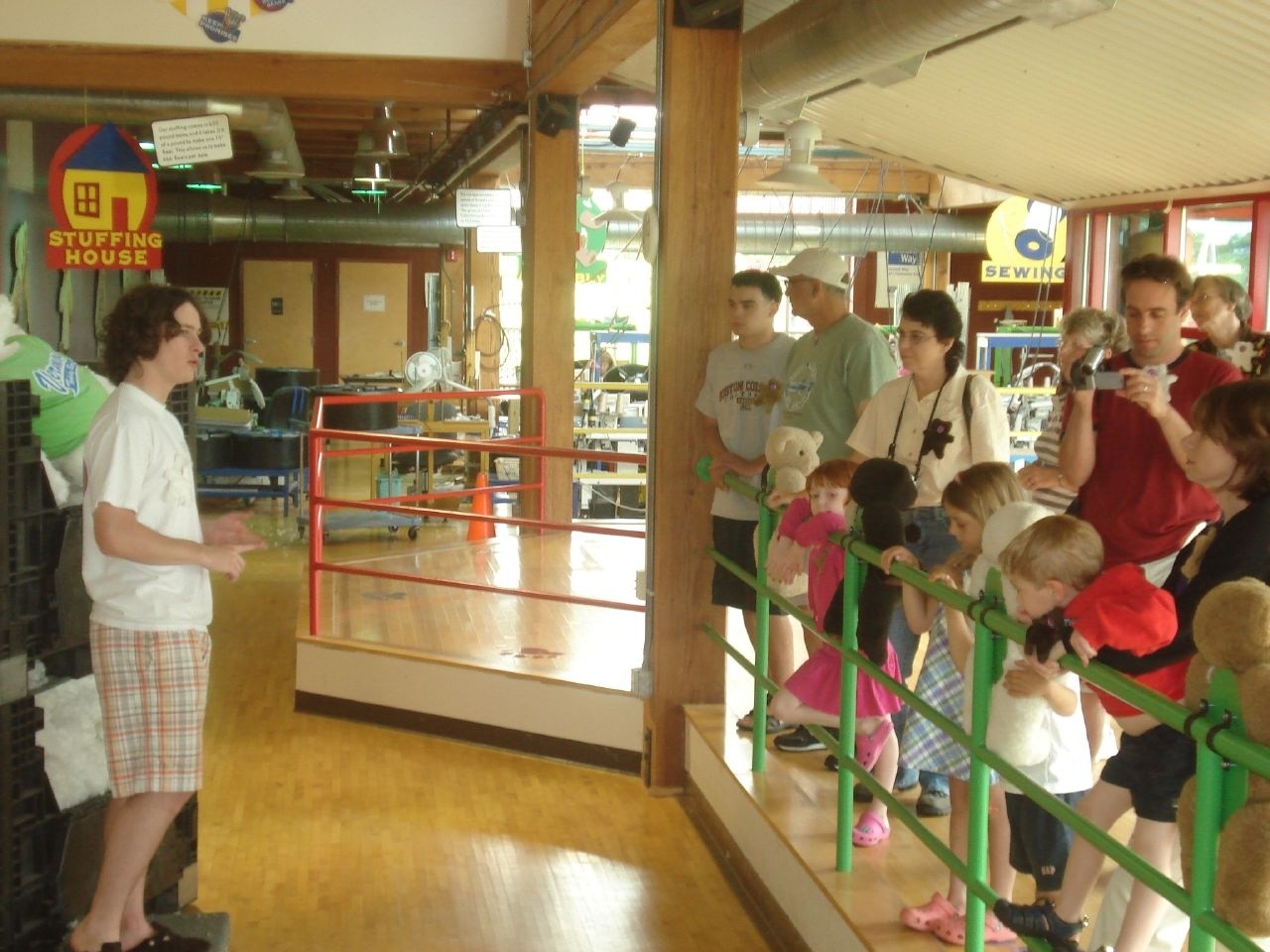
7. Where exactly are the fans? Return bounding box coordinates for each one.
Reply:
[404,351,441,389]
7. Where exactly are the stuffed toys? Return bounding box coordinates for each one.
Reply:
[1180,579,1270,937]
[752,425,824,599]
[823,457,918,666]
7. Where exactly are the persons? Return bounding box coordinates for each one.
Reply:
[764,457,900,847]
[774,247,897,774]
[897,461,1027,944]
[59,285,269,951]
[980,252,1269,952]
[852,290,1011,816]
[693,270,800,736]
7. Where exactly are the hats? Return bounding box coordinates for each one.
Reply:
[768,246,851,291]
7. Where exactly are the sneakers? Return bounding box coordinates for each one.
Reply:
[826,753,840,771]
[916,788,951,816]
[774,724,840,751]
[853,782,874,802]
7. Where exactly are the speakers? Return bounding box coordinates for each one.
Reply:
[535,102,570,138]
[610,117,636,147]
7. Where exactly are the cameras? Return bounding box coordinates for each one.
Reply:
[1069,346,1126,392]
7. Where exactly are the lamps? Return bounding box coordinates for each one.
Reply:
[196,357,266,409]
[755,120,839,193]
[353,104,409,159]
[352,182,388,196]
[353,157,390,183]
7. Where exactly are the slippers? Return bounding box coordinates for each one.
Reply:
[900,893,960,933]
[933,908,1019,945]
[852,811,890,846]
[855,719,893,773]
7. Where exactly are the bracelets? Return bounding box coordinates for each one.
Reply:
[1056,467,1065,486]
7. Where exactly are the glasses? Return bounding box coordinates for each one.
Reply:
[1190,293,1226,310]
[785,278,818,290]
[896,326,943,345]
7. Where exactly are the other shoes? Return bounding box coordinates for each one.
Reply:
[129,922,209,952]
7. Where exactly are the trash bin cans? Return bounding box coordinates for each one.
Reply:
[375,476,402,498]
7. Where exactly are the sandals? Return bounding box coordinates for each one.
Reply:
[993,897,1089,952]
[736,693,800,732]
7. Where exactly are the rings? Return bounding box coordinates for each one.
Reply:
[1144,383,1147,392]
